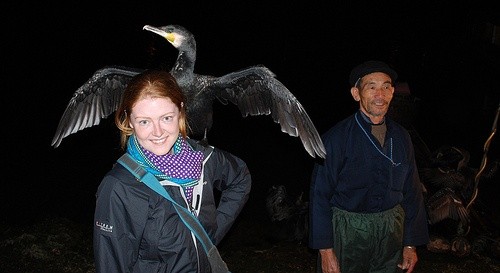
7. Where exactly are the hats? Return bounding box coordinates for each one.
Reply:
[350,60,397,86]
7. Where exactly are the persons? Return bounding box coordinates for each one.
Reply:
[93,69,251,273]
[309,62,429,273]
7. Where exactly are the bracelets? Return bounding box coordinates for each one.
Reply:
[407,245,416,251]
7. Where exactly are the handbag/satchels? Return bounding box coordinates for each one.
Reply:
[416,154,450,191]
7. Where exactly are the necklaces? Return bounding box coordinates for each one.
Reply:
[355,112,401,168]
[369,121,384,126]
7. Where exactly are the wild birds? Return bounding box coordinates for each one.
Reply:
[50,24,327,161]
[268,184,311,246]
[414,142,496,239]
